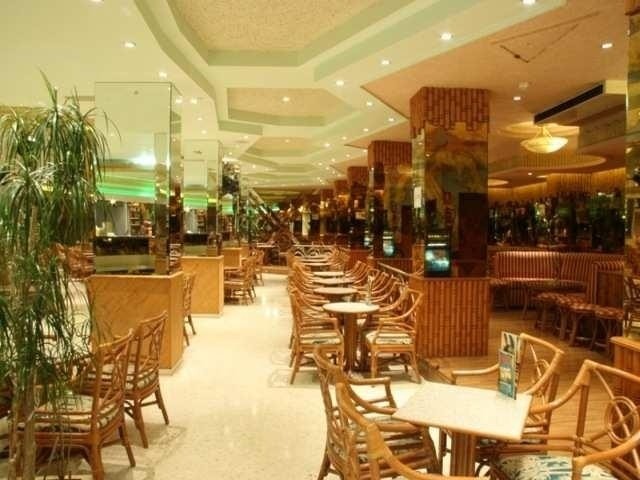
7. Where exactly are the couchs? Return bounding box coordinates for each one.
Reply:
[491,250,626,305]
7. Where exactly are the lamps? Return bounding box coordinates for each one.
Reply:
[520,126,569,153]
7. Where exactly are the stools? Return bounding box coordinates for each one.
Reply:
[590,309,624,358]
[491,279,510,311]
[569,304,599,347]
[533,293,565,333]
[553,297,584,340]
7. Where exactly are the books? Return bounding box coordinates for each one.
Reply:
[496,350,515,397]
[499,329,522,365]
[499,365,513,395]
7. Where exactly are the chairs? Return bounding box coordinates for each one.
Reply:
[441,332,565,476]
[72,309,169,448]
[335,378,474,480]
[521,283,548,319]
[9,328,136,480]
[224,260,254,305]
[184,271,196,346]
[626,275,640,336]
[287,248,423,385]
[313,343,427,480]
[474,359,640,480]
[228,257,256,298]
[255,251,264,286]
